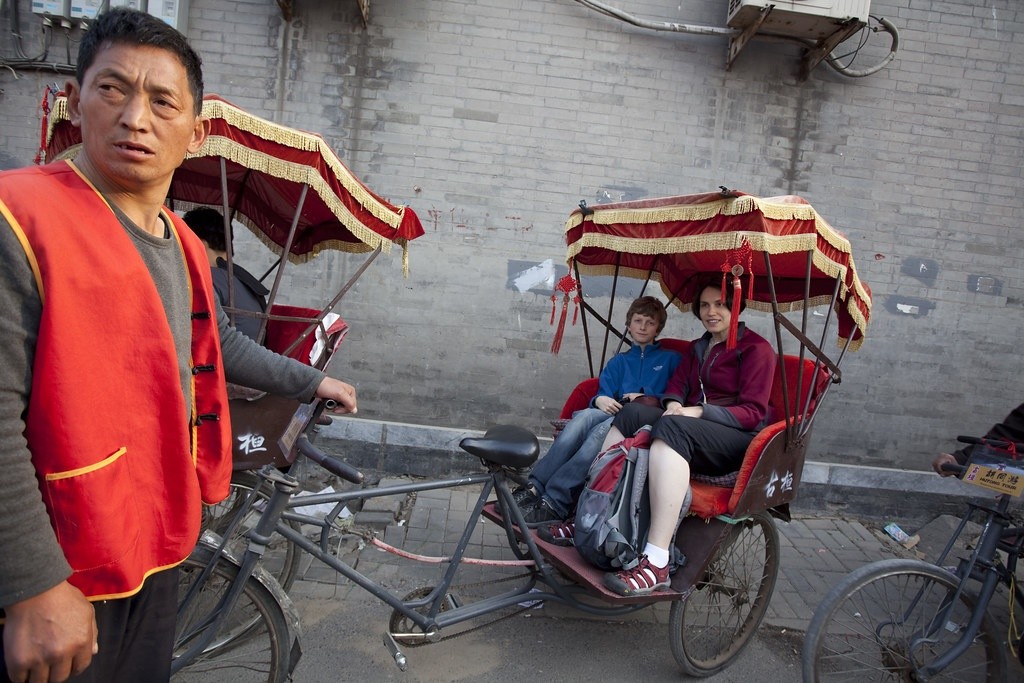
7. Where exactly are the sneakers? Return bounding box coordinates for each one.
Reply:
[510,496,562,529]
[494,486,536,514]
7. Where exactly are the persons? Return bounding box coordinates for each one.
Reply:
[934,403,1024,477]
[537,280,776,595]
[496,297,680,529]
[0,9,357,683]
[183,206,269,346]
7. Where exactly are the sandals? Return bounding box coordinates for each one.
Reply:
[538,516,576,547]
[604,554,671,596]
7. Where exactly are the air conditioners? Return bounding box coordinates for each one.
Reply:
[726,0,871,43]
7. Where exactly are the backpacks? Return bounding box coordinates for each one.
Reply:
[574,423,692,570]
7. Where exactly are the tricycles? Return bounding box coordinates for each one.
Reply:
[13,84,424,667]
[168,187,872,683]
[800,433,1024,683]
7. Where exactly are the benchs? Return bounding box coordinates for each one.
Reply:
[261,303,345,366]
[552,337,830,518]
